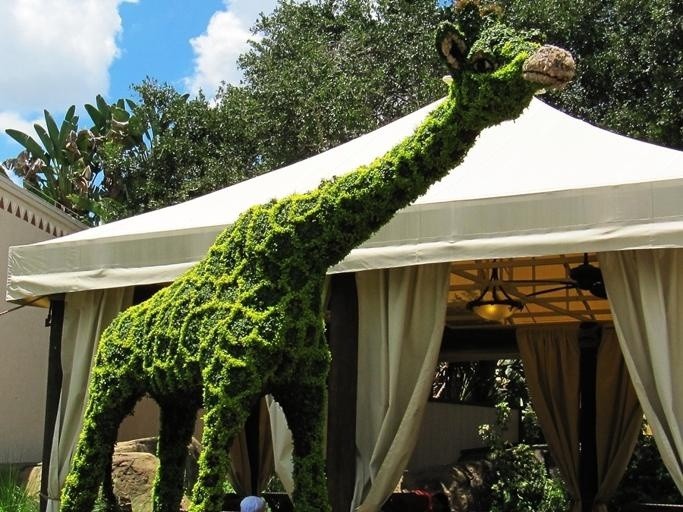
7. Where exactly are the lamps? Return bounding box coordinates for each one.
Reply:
[467,259,523,322]
